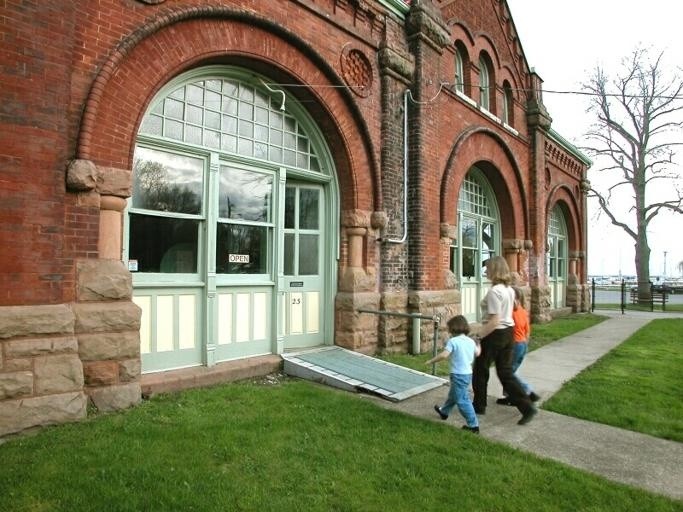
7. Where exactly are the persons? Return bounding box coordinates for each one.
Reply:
[495,286,541,406]
[471,256,538,425]
[423,315,481,435]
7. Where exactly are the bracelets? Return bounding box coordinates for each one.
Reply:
[476,334,481,341]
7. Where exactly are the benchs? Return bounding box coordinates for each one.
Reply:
[630,287,668,305]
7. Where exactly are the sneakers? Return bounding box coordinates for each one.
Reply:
[517,405,539,426]
[434,405,449,420]
[470,391,540,415]
[460,424,480,435]
